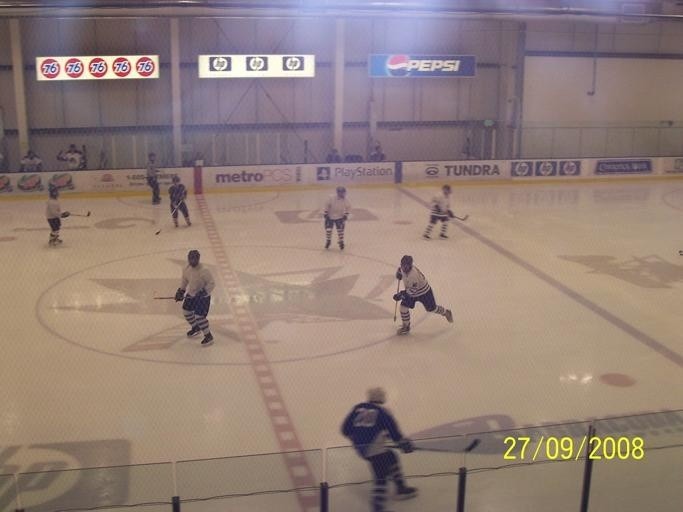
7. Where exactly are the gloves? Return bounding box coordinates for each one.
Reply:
[176,288,186,299]
[396,268,403,279]
[197,289,206,296]
[400,442,413,453]
[393,292,405,301]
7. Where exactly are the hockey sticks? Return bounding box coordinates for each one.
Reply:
[70,211,91,216]
[453,215,469,221]
[155,200,182,234]
[385,438,481,453]
[151,290,189,299]
[393,279,400,321]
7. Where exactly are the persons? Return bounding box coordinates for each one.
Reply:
[44,187,70,241]
[422,184,456,240]
[324,186,351,249]
[99,150,107,169]
[393,255,454,334]
[20,151,42,172]
[174,250,216,344]
[146,153,163,203]
[341,386,420,512]
[326,147,343,163]
[168,175,192,227]
[61,144,85,171]
[368,142,385,162]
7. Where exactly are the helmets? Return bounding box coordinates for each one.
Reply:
[189,251,199,258]
[337,185,347,193]
[443,185,451,193]
[368,387,386,403]
[402,257,413,267]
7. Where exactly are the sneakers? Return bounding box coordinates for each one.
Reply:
[339,242,344,248]
[201,333,212,343]
[446,310,451,320]
[440,234,447,239]
[399,485,415,495]
[187,325,200,335]
[423,234,431,239]
[398,327,410,332]
[325,241,330,248]
[49,238,62,247]
